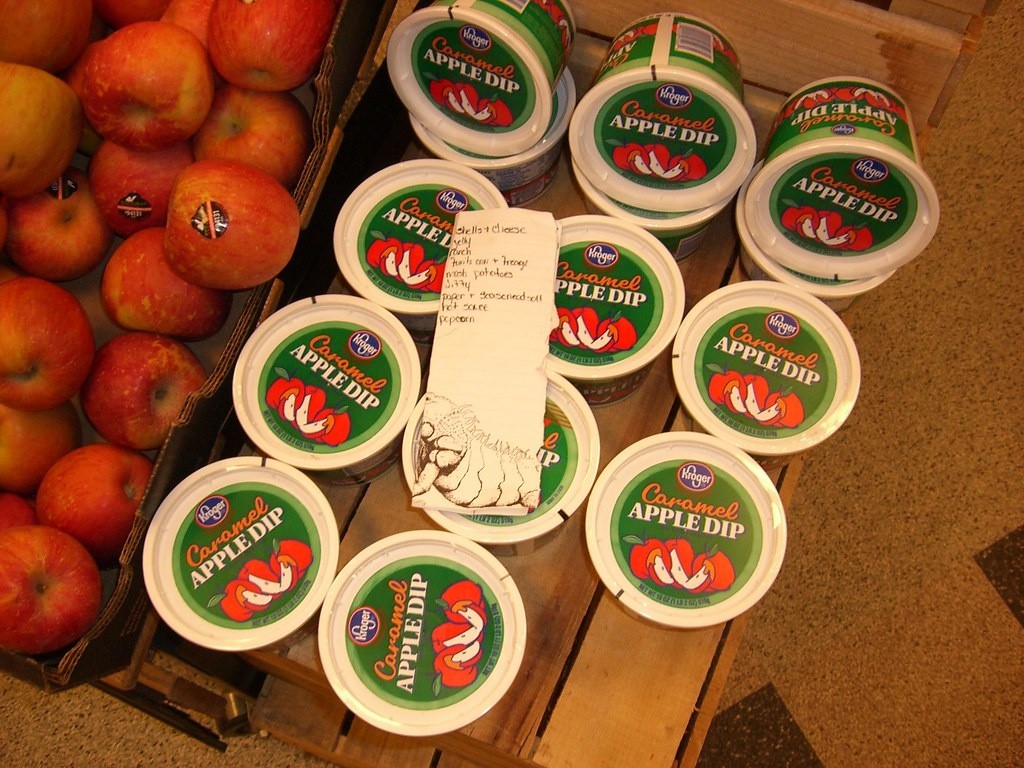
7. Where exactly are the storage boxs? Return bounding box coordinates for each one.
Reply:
[0,0,1000,768]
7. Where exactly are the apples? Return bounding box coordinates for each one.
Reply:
[0,0,333,658]
